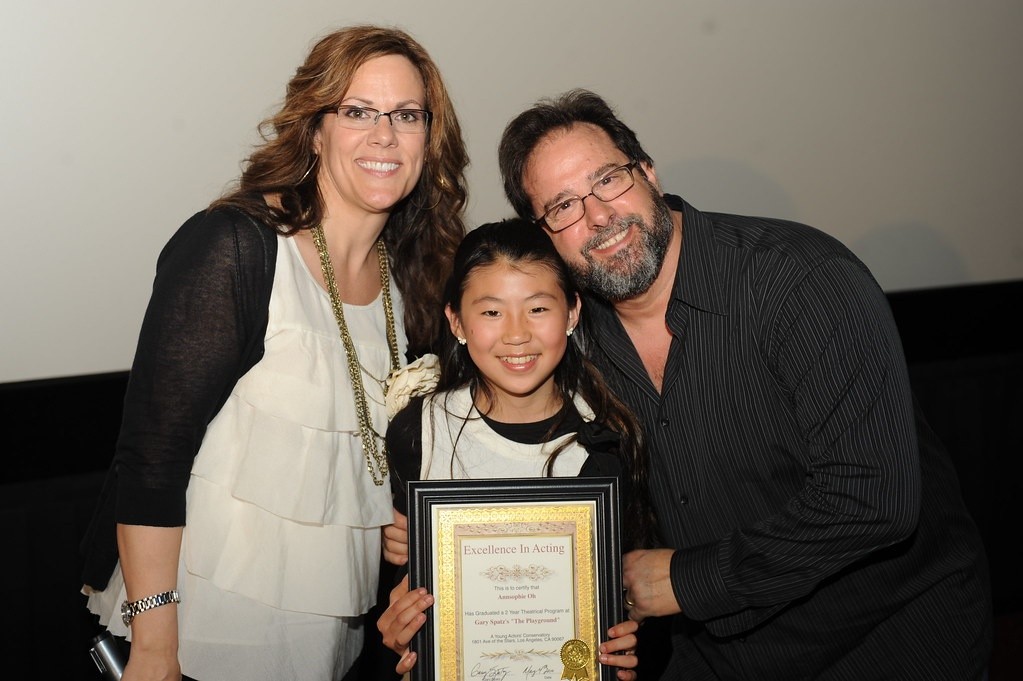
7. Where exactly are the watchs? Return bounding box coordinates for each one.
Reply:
[120,590,181,628]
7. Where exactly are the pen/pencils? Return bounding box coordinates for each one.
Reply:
[89,632,126,681]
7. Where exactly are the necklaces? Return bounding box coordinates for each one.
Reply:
[304,209,400,487]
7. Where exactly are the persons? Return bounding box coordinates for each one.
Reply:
[381,89,1002,681]
[375,217,644,681]
[73,26,470,681]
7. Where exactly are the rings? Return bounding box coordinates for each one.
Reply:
[624,596,634,606]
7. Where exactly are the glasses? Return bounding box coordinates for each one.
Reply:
[531,157,638,234]
[321,105,433,133]
